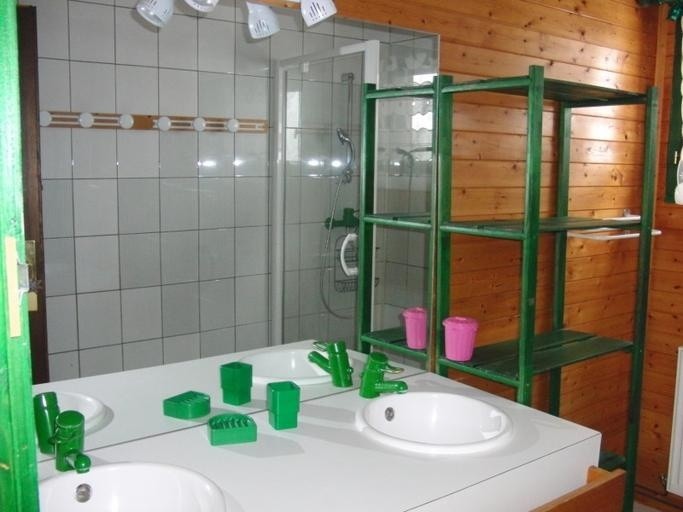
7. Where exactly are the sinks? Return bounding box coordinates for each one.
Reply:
[236,348,349,388]
[355,391,516,456]
[36,461,226,512]
[33,389,112,450]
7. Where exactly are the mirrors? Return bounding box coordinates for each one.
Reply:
[18,0,440,463]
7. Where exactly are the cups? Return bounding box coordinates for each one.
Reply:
[441,315,479,362]
[267,381,301,431]
[403,308,426,350]
[220,362,252,406]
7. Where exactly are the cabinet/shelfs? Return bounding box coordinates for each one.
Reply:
[437,65,659,511]
[357,76,437,370]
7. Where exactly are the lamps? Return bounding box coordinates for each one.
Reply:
[184,0,220,13]
[287,0,337,27]
[246,1,280,39]
[136,0,175,28]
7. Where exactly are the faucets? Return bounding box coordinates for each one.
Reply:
[359,351,409,398]
[33,391,62,456]
[307,339,354,388]
[48,409,92,473]
